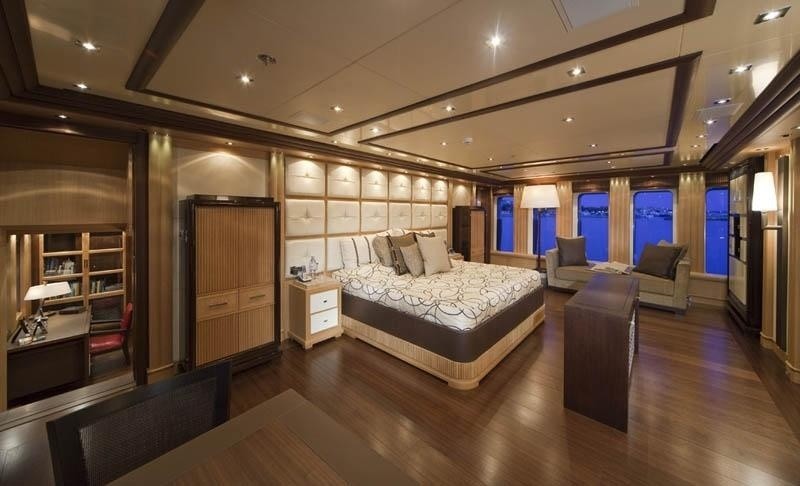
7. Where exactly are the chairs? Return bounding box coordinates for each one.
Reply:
[89,302,134,377]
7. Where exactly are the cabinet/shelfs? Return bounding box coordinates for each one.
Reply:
[179,193,282,385]
[39,231,127,329]
[453,206,492,264]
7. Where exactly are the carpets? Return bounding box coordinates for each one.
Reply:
[105,388,422,486]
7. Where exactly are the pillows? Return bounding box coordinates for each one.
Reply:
[656,240,689,279]
[556,235,589,266]
[632,243,682,281]
[340,228,451,278]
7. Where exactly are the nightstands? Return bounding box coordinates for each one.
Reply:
[451,256,464,260]
[288,275,342,350]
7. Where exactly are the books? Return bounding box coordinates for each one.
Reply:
[584,262,634,275]
[44,257,123,300]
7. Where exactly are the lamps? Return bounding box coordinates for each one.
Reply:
[751,172,783,230]
[520,185,561,273]
[23,281,73,317]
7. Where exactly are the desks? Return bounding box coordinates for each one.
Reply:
[7,304,92,408]
[564,272,639,434]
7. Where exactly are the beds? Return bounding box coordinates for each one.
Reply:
[331,259,547,391]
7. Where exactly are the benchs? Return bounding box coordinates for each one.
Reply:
[545,247,690,314]
